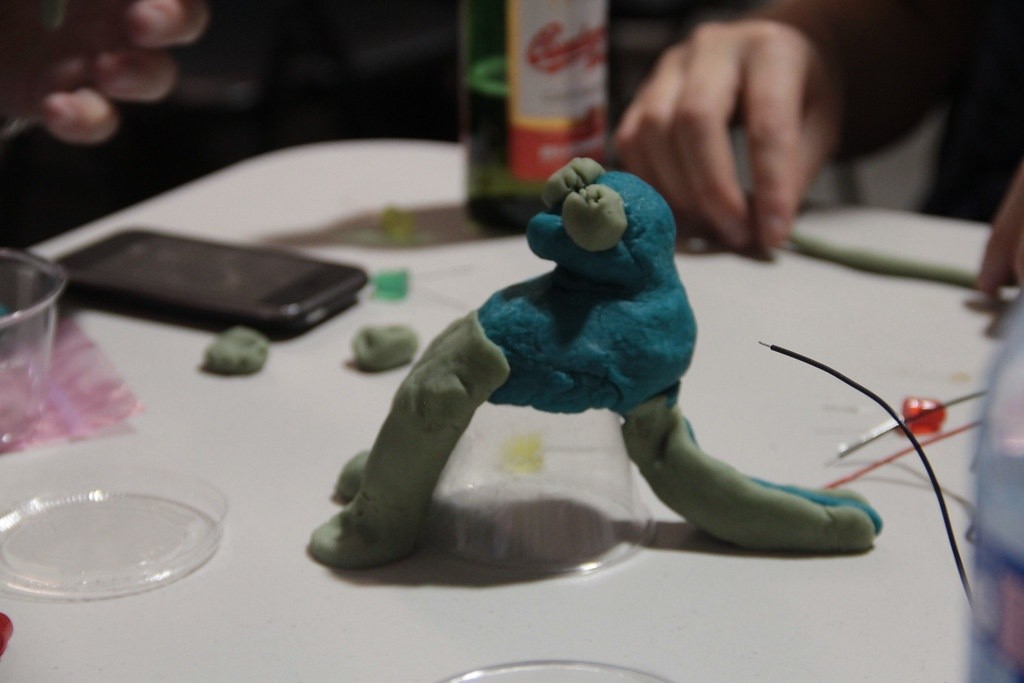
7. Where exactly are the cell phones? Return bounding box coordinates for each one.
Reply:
[36,230,367,339]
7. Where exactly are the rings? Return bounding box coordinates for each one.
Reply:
[42,3,65,28]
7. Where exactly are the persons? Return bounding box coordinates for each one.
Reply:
[611,0,1024,305]
[0,0,208,144]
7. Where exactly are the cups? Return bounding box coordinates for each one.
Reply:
[0,246,69,453]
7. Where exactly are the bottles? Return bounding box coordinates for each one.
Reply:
[960,296,1024,683]
[455,0,611,235]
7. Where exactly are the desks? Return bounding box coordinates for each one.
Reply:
[0,140,1024,683]
